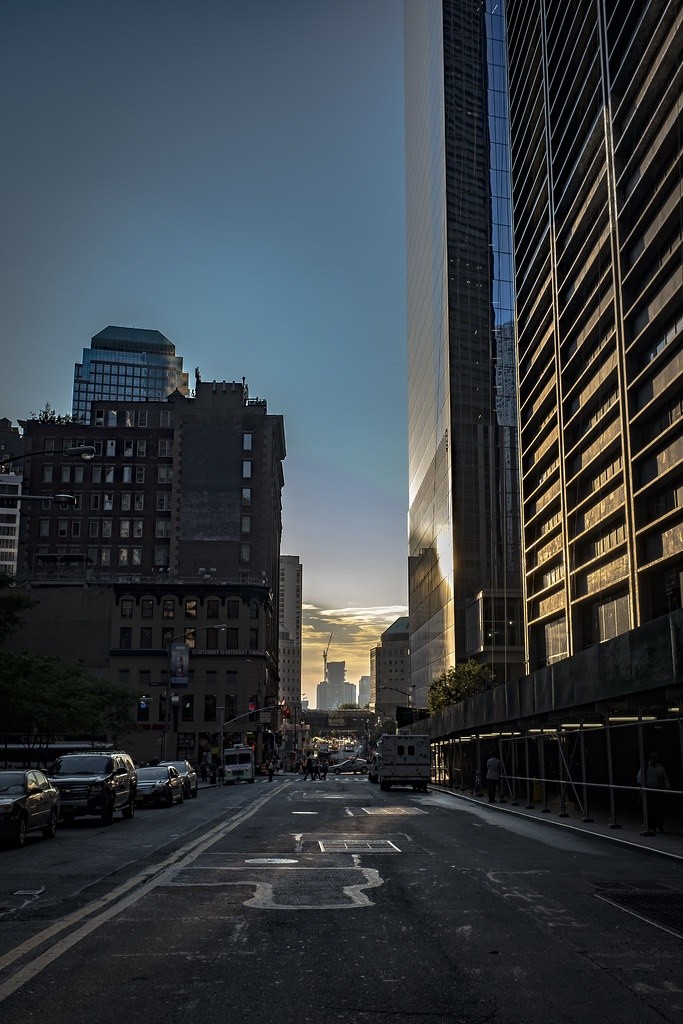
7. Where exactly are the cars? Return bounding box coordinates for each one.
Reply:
[48,750,138,826]
[327,755,368,775]
[0,766,62,848]
[135,758,200,809]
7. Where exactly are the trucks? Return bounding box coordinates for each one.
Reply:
[366,733,432,792]
[221,742,257,785]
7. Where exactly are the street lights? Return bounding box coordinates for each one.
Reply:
[0,442,100,508]
[379,683,410,710]
[163,621,229,767]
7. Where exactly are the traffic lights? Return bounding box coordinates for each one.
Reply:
[247,695,261,722]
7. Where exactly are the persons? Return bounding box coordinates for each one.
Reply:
[636,753,670,833]
[199,761,209,783]
[486,751,501,803]
[268,759,275,781]
[134,756,160,769]
[303,758,328,781]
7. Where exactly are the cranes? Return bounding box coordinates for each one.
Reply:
[321,631,334,686]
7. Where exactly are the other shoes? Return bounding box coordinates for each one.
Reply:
[488,799,497,802]
[651,824,666,833]
